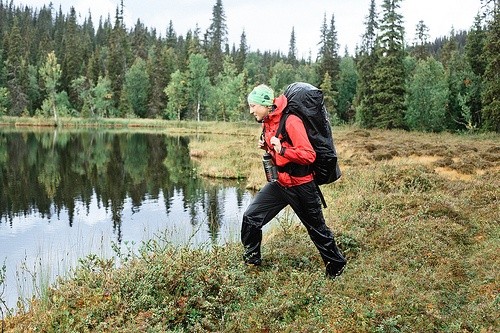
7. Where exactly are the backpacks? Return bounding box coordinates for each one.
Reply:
[259,82,342,184]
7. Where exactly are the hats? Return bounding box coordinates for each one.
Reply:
[248,83,275,106]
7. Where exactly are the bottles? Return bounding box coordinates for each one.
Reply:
[262,153,278,182]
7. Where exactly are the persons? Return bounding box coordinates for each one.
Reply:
[237,84,348,281]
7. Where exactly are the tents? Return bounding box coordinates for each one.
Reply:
[283,81,343,185]
